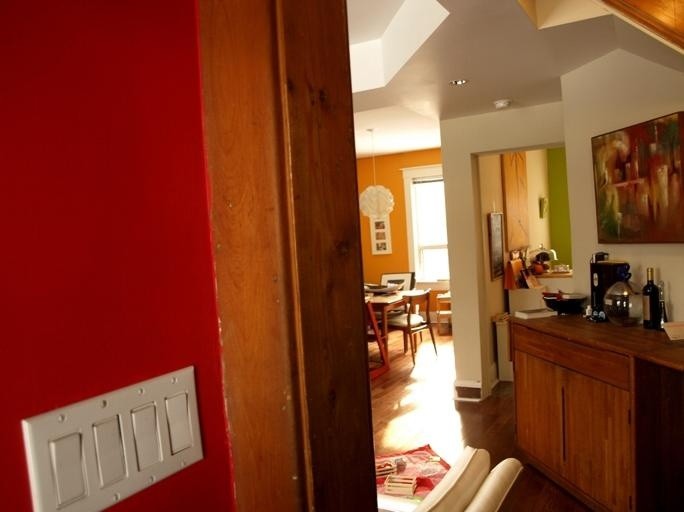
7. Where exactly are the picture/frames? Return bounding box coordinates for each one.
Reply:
[591,111,684,244]
[369,215,392,255]
[488,212,504,281]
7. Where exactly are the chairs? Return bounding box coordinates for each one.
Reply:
[363,279,439,368]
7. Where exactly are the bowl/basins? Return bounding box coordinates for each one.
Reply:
[542,295,587,311]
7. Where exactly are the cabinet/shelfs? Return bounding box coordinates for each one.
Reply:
[506,309,682,511]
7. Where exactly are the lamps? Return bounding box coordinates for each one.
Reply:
[358,128,396,219]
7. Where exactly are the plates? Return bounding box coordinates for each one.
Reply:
[364,285,402,293]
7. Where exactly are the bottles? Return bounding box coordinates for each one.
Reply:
[585,305,592,316]
[642,267,667,332]
